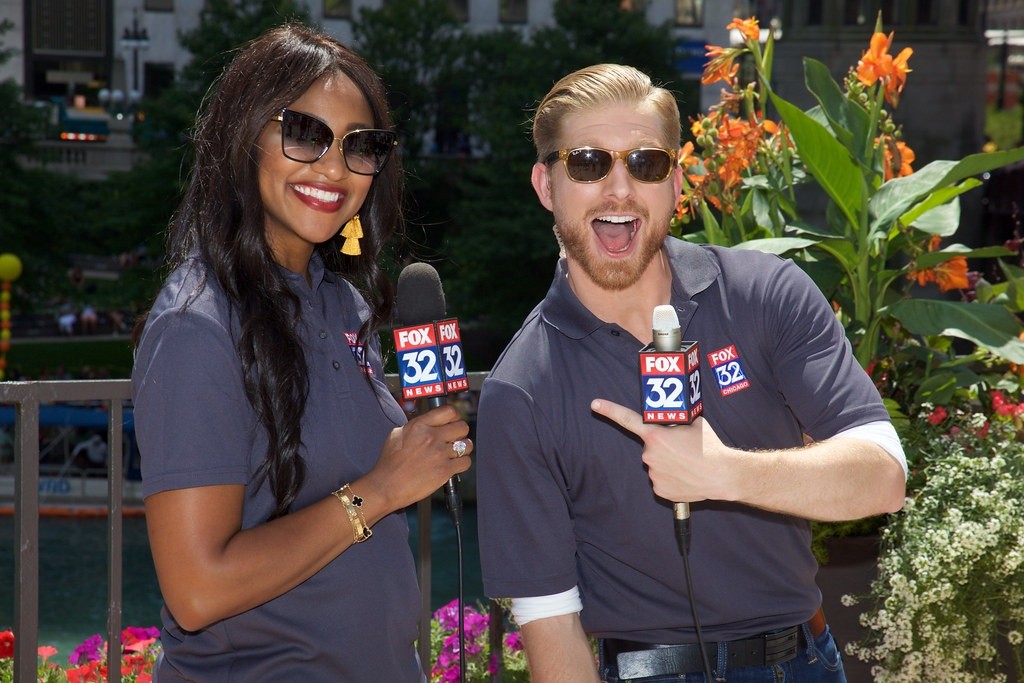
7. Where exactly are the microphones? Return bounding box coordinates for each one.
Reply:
[639,304,703,557]
[393,263,471,527]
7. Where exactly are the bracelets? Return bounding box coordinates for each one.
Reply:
[330,481,373,542]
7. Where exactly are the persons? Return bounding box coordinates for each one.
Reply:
[70,433,108,475]
[476,61,909,682]
[15,252,138,332]
[3,364,114,409]
[131,20,475,683]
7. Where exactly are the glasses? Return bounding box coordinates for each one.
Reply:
[535,145,684,184]
[269,107,398,179]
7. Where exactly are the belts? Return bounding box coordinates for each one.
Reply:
[601,603,839,678]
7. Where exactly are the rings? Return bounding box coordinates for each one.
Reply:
[452,440,466,456]
